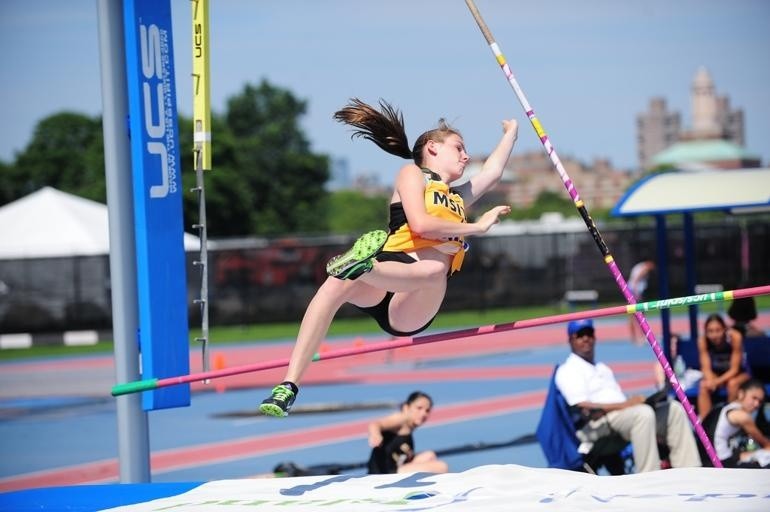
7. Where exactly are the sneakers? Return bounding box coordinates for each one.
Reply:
[326,229,389,280]
[258,381,299,418]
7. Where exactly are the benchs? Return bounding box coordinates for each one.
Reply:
[645,334,769,440]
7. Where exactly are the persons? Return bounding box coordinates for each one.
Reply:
[259,98,517,418]
[367,393,448,473]
[696,314,753,421]
[699,378,770,469]
[626,259,656,346]
[728,297,765,337]
[653,335,685,391]
[554,318,703,474]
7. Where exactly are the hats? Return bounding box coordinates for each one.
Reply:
[567,318,594,336]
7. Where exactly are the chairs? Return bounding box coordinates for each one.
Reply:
[535,365,630,477]
[696,406,770,468]
